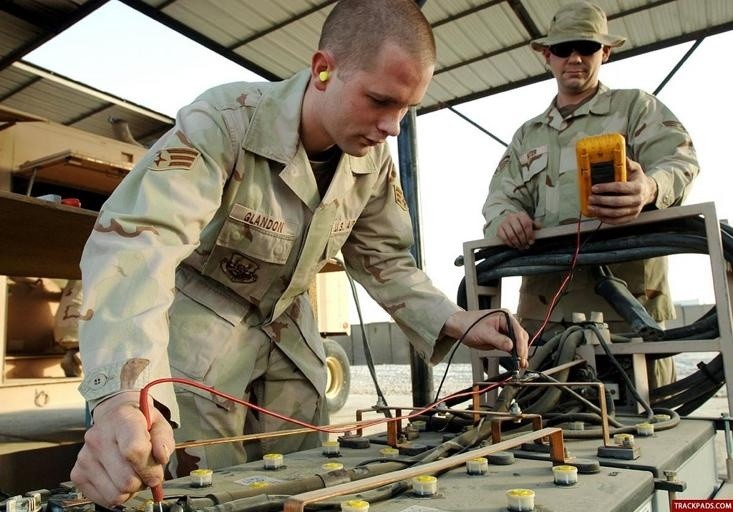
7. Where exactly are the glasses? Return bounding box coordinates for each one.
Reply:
[551,41,601,56]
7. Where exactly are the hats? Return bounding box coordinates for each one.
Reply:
[532,3,626,51]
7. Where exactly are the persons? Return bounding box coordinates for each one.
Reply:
[482,1,699,411]
[70,0,529,509]
